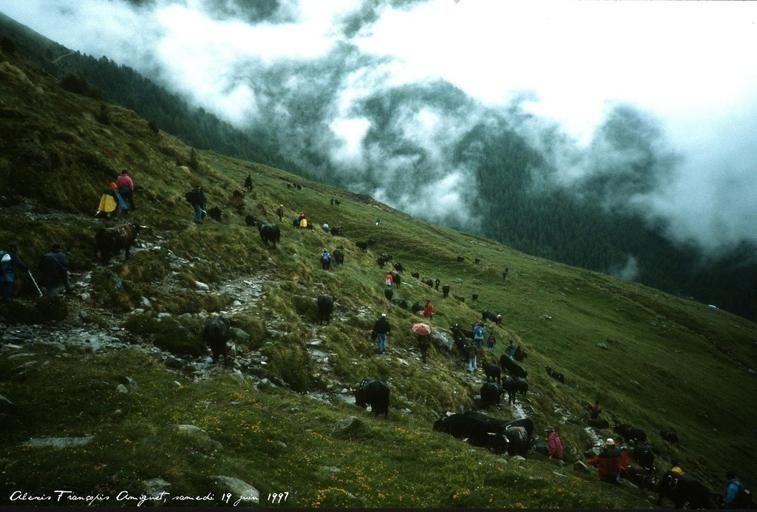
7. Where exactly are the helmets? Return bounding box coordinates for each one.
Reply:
[606,437,616,445]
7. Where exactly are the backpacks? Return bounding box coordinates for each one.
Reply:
[738,485,752,508]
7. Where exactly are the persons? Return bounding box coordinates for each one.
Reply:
[277,182,527,373]
[657,466,683,505]
[190,185,207,224]
[94,168,138,221]
[718,471,744,511]
[1,241,30,304]
[544,400,628,483]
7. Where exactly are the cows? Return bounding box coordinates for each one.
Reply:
[431,412,494,438]
[545,365,564,383]
[317,293,335,325]
[478,352,530,405]
[96,221,151,266]
[353,377,390,419]
[632,446,656,466]
[656,480,714,510]
[611,423,647,442]
[203,314,232,367]
[258,222,282,248]
[119,236,122,238]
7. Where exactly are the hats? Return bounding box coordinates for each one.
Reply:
[672,466,683,475]
[110,182,119,190]
